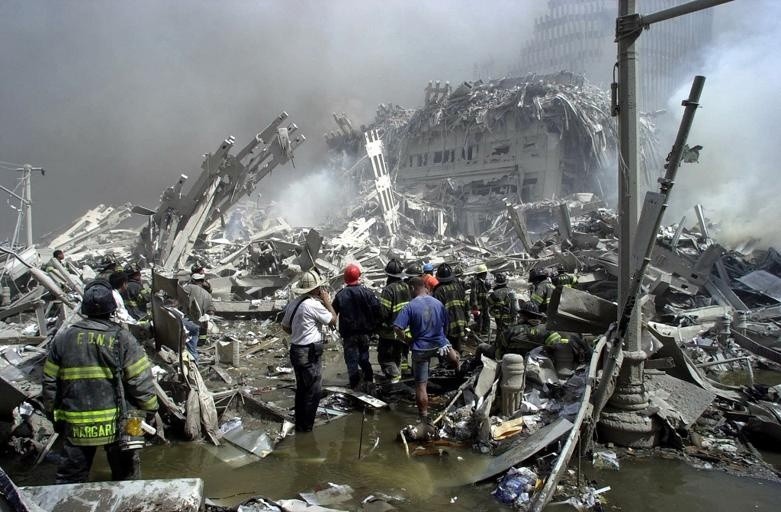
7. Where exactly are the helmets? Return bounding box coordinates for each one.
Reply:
[82,285,117,315]
[344,265,361,285]
[99,254,141,275]
[292,271,325,294]
[384,259,508,285]
[518,301,539,316]
[529,266,564,282]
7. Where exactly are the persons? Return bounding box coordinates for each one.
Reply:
[332,258,574,417]
[279,269,337,432]
[40,279,162,484]
[45,249,213,365]
